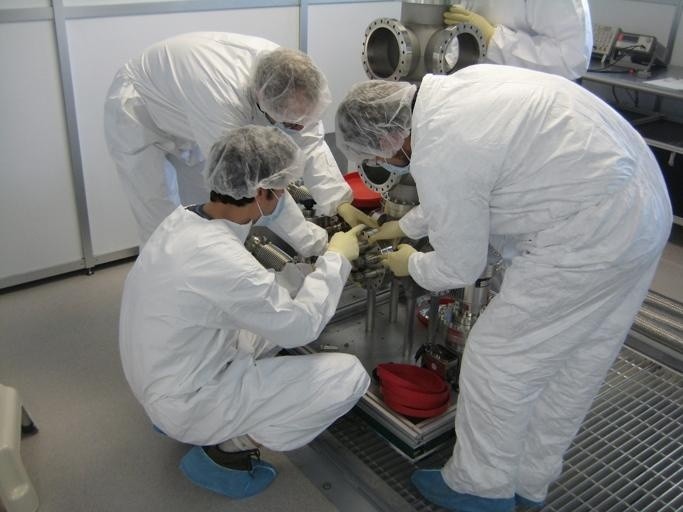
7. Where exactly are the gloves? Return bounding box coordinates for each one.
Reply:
[327,224,366,261]
[378,244,419,277]
[336,201,378,230]
[442,4,497,46]
[368,220,406,250]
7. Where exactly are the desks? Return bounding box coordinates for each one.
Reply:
[575,56,683,229]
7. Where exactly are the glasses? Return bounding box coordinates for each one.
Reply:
[366,157,379,169]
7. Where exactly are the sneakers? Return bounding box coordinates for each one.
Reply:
[410,468,516,512]
[177,444,278,499]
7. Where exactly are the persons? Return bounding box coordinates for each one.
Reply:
[444,0,593,81]
[102,31,379,257]
[333,62,674,512]
[120,124,372,498]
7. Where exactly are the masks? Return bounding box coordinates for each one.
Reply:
[377,146,411,175]
[250,190,287,227]
[275,122,306,136]
